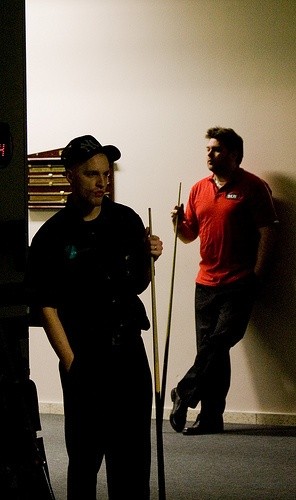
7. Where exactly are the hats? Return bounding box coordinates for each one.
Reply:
[62,135,122,171]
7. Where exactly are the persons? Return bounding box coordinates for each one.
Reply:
[29,135,163,500]
[169,126,278,436]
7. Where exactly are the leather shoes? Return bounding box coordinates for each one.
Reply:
[184,414,225,436]
[169,389,188,432]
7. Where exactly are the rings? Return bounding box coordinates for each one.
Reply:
[154,245,157,250]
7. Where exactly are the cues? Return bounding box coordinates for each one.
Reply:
[148,182,182,500]
